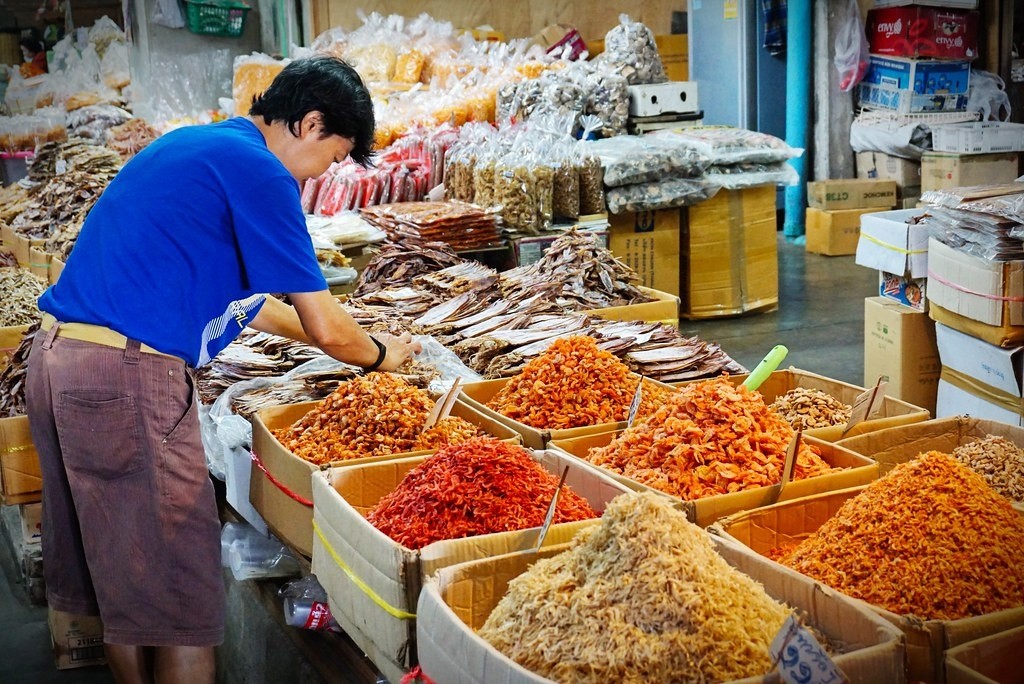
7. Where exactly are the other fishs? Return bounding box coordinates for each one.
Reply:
[0,116,166,419]
[194,240,747,426]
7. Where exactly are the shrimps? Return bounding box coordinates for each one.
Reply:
[273,328,1024,683]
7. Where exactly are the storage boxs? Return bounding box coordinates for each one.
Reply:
[4,8,1024,684]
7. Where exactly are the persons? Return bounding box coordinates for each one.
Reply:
[26,46,424,683]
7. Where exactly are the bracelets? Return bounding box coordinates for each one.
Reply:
[363,333,388,373]
